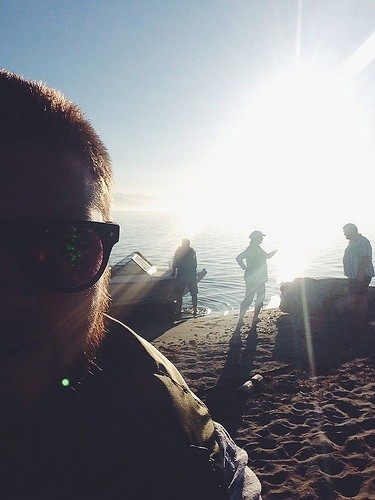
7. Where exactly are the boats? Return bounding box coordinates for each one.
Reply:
[108,250,208,319]
[279,277,375,315]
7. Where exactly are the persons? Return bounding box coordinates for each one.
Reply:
[343,222,375,295]
[172,238,199,318]
[236,230,277,327]
[1,70,261,499]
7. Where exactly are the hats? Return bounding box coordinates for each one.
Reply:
[249,231,267,239]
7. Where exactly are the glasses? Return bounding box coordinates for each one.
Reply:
[0,218,120,294]
